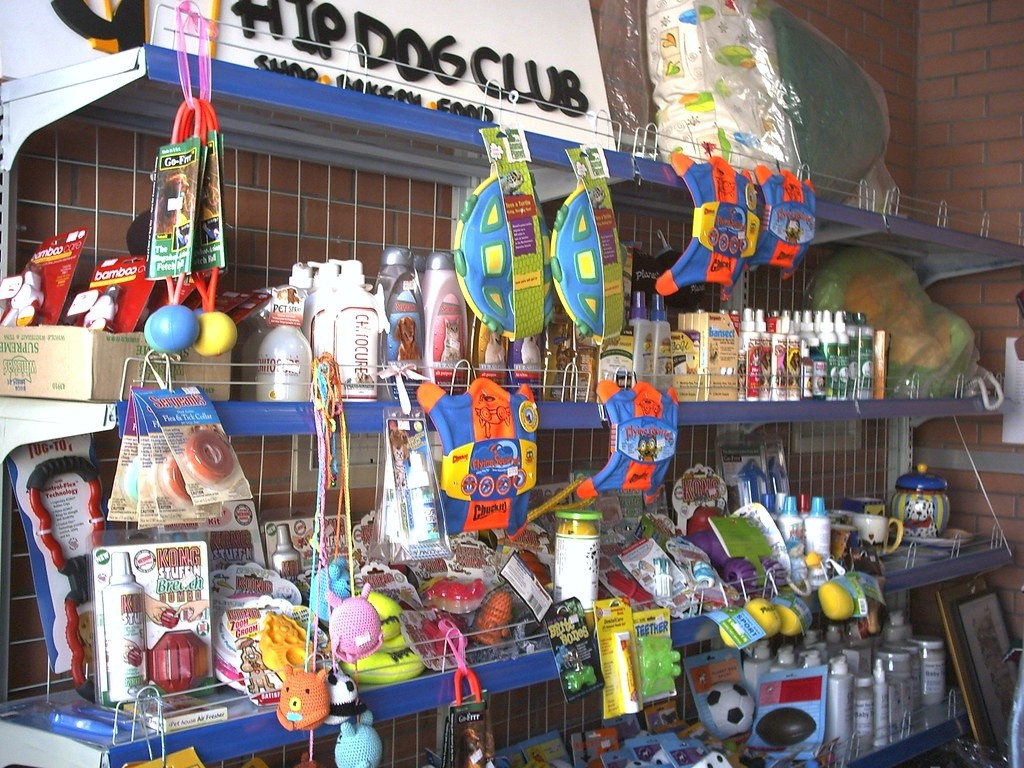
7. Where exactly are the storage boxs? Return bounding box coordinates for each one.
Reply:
[0,324,232,404]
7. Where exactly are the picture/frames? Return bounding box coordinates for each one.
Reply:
[936,583,1020,765]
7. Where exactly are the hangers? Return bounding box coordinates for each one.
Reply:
[656,228,670,250]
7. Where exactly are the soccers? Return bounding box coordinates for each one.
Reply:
[705,681,756,736]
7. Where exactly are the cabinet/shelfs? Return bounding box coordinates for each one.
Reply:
[0,0,1024,768]
